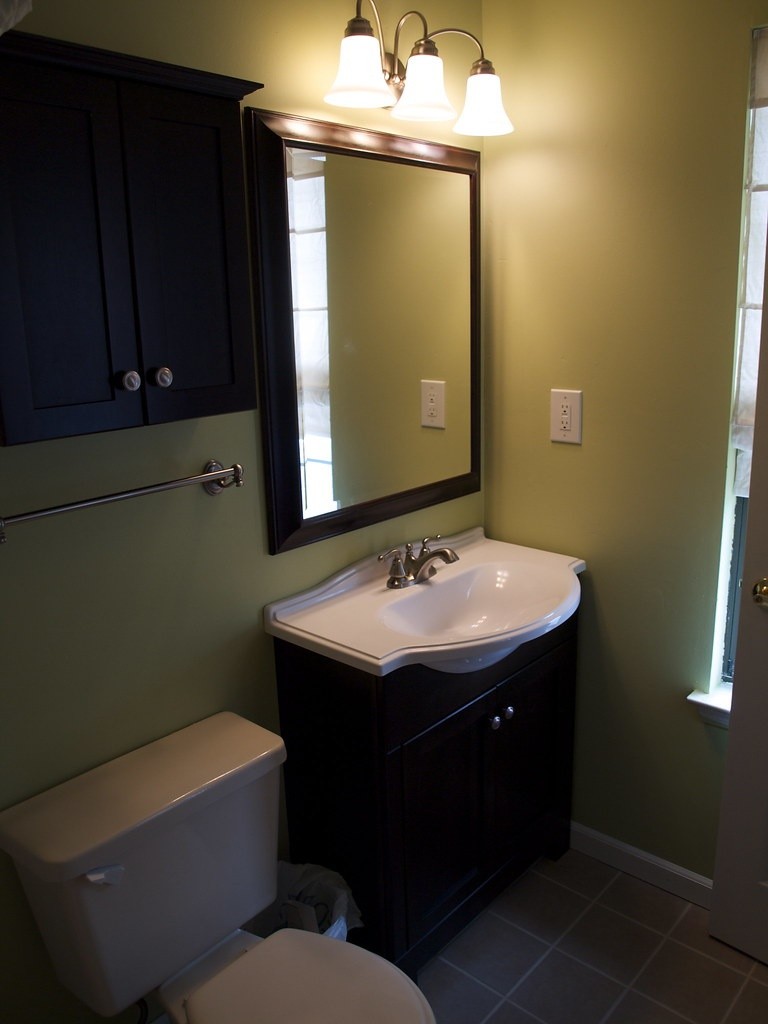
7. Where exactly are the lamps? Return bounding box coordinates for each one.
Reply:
[325,0,515,139]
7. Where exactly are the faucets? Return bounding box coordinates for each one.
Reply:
[405,544,459,582]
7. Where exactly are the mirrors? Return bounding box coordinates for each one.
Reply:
[243,106,483,556]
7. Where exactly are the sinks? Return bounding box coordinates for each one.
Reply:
[262,527,586,677]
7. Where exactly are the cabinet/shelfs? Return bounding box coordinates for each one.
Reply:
[0,29,264,447]
[274,600,581,988]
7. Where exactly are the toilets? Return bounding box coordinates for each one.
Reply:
[0,712,438,1024]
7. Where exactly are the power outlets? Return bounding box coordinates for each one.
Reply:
[422,380,446,430]
[549,388,583,447]
